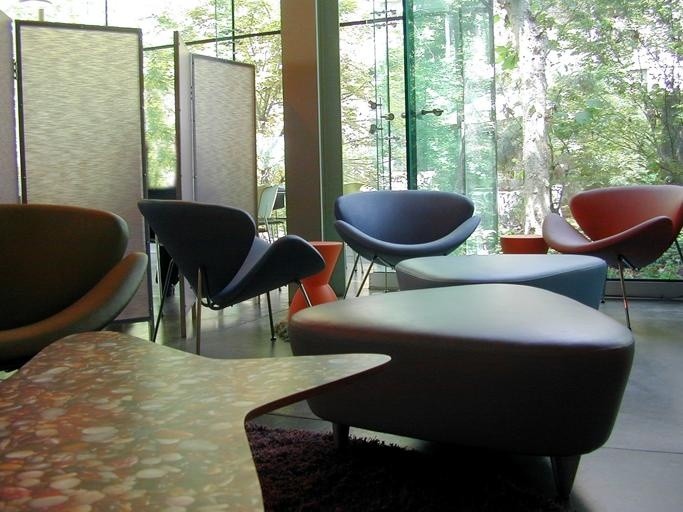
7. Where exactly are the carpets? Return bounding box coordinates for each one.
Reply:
[246,425,579,512]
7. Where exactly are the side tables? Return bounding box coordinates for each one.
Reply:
[288,242,339,321]
[500,236,547,254]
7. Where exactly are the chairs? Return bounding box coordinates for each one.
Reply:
[333,190,482,300]
[257,184,286,245]
[0,204,150,372]
[543,185,683,331]
[137,199,326,355]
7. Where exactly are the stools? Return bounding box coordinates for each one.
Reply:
[395,254,607,310]
[289,283,633,503]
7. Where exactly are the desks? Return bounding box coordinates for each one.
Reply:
[0,332,392,512]
